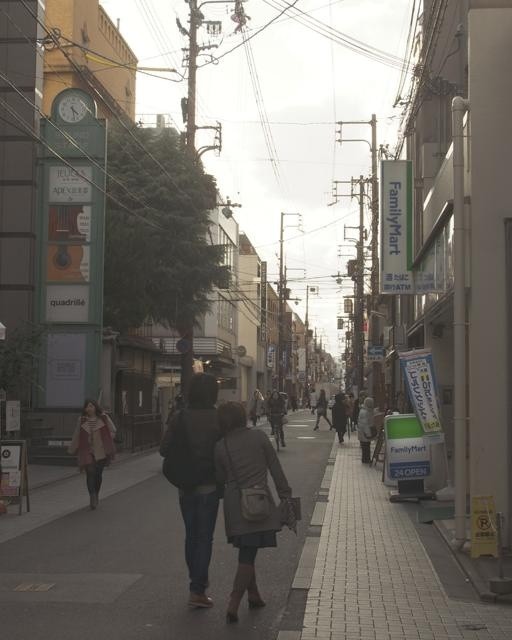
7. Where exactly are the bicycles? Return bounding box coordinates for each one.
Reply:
[267,415,284,448]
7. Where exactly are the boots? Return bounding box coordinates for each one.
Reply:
[225,561,267,624]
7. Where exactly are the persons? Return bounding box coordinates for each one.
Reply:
[156,370,227,608]
[248,384,365,450]
[212,399,296,624]
[357,397,379,464]
[70,399,117,511]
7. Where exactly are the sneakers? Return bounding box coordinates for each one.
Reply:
[187,591,215,609]
[90,494,99,509]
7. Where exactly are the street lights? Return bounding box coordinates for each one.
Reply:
[336,295,364,386]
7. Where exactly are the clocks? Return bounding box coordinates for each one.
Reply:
[51,90,92,126]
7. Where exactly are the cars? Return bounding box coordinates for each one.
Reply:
[328,395,335,409]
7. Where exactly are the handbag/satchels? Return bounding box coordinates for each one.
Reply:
[279,495,302,525]
[363,426,377,440]
[237,487,273,523]
[161,411,202,492]
